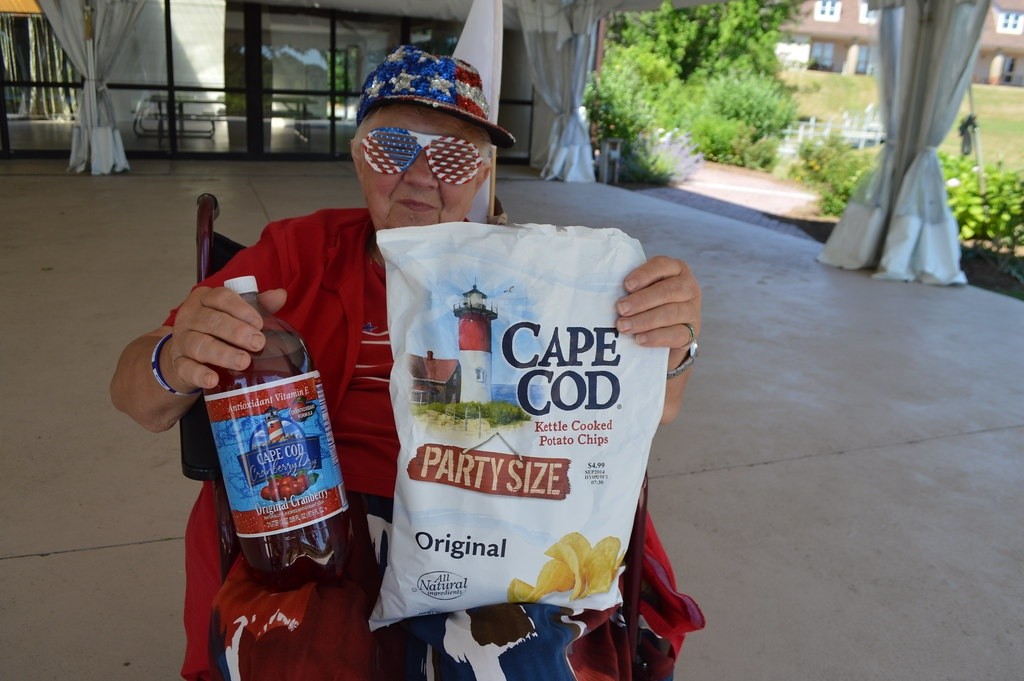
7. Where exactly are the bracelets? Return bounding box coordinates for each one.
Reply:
[151,332,203,397]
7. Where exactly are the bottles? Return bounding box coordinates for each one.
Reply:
[203,274,350,591]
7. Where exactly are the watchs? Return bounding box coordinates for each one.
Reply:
[666,338,698,378]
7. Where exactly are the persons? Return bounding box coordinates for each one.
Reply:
[109,44,702,681]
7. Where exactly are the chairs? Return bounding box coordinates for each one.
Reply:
[174,193,652,681]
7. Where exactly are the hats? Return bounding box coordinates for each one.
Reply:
[356,44,516,148]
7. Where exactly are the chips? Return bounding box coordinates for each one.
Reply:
[507,532,626,603]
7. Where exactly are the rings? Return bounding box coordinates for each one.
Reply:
[681,323,695,348]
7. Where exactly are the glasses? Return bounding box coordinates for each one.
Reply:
[354,128,484,184]
